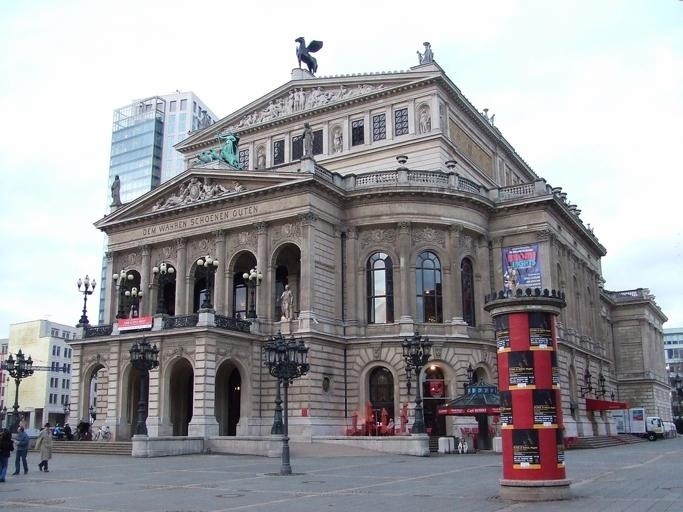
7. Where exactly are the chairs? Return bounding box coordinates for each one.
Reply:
[346,417,395,436]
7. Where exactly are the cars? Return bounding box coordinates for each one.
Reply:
[39,426,72,440]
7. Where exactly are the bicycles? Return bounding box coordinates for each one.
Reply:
[73,425,113,440]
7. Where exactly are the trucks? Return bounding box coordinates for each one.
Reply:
[607,407,663,441]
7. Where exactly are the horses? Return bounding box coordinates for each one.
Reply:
[294,35,324,75]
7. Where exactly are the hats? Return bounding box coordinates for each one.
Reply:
[44,423,50,427]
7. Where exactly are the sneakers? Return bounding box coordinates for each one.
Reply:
[37,464,42,471]
[12,472,19,475]
[23,467,28,474]
[0,479,4,482]
[43,470,49,472]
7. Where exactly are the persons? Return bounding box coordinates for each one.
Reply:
[194,130,240,167]
[35,423,53,472]
[11,426,29,475]
[279,285,294,320]
[458,438,468,454]
[111,175,121,204]
[53,424,63,441]
[63,424,73,440]
[295,121,314,155]
[387,418,394,430]
[0,427,14,482]
[416,41,434,65]
[504,266,520,294]
[76,420,89,439]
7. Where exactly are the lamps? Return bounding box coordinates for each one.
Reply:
[595,372,606,398]
[405,364,412,395]
[465,363,474,384]
[610,389,615,402]
[581,369,592,398]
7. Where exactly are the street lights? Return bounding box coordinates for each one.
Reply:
[403,331,433,434]
[262,329,285,434]
[1,346,34,432]
[76,274,95,323]
[241,266,262,318]
[196,254,220,308]
[112,268,134,319]
[152,261,174,313]
[267,334,308,474]
[129,332,160,434]
[123,286,143,318]
